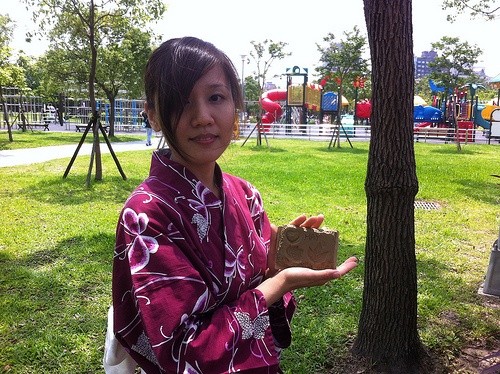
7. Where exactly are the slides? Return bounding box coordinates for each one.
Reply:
[415,103,435,126]
[257,88,287,133]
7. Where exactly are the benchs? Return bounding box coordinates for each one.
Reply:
[75,124,111,133]
[17,122,50,131]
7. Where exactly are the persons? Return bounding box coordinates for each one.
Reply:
[106,37,359,373]
[140,109,152,146]
